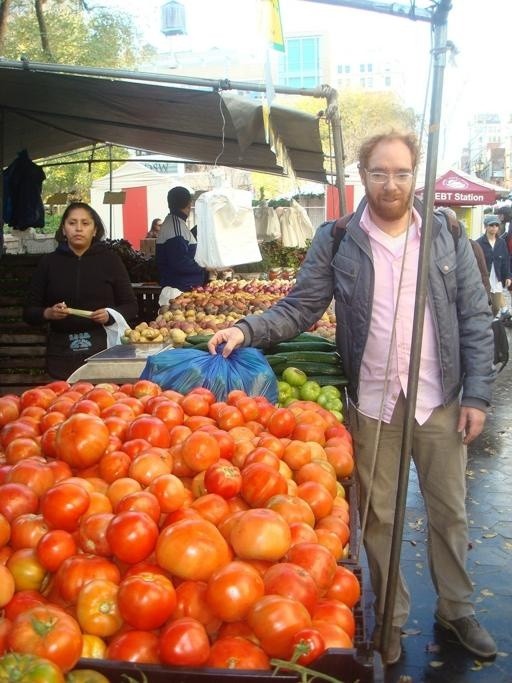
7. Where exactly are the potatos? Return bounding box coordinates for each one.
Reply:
[120,279,336,342]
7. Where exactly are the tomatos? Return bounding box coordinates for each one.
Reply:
[2,380,358,683]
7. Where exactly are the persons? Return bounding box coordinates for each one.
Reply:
[207,128,497,666]
[154,186,209,306]
[147,218,163,238]
[21,202,139,382]
[437,206,512,317]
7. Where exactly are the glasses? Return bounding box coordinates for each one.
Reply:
[364,166,416,183]
[490,224,499,227]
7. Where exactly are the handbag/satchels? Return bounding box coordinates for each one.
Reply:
[57,326,107,373]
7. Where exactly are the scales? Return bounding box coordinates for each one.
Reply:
[64,343,177,387]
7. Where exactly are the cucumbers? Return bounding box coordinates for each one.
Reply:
[183,330,349,386]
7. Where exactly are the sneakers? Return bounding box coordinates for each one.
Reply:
[434,610,498,657]
[371,624,401,664]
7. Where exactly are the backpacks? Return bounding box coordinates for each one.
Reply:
[491,320,509,364]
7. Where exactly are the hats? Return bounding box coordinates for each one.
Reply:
[484,215,501,225]
